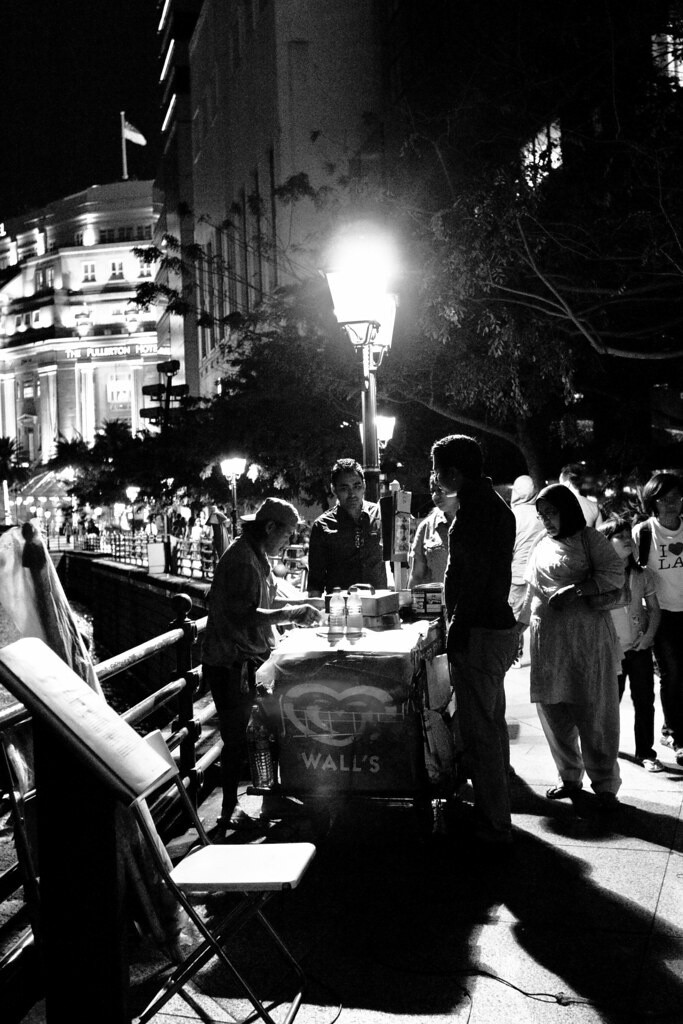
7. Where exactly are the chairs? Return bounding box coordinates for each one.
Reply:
[127,727,316,1024]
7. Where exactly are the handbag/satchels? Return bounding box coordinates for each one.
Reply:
[579,529,632,610]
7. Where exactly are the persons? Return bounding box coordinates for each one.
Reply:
[202,498,323,831]
[431,431,521,834]
[512,484,625,809]
[62,458,683,772]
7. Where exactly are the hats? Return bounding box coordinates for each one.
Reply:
[239,496,301,527]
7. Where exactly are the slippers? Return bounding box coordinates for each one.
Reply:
[217,814,257,829]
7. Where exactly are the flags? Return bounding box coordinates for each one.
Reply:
[123,118,147,145]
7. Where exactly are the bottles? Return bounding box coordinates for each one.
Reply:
[329,587,344,633]
[347,588,363,634]
[246,704,274,787]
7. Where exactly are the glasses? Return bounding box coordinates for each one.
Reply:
[537,512,553,521]
[654,494,683,507]
[354,526,365,548]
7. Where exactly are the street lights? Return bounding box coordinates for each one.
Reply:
[326,235,396,501]
[126,486,139,557]
[44,509,52,549]
[221,456,246,539]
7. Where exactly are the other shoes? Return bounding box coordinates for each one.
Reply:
[637,755,664,772]
[593,792,614,810]
[546,781,582,799]
[673,744,683,759]
[663,735,674,747]
[462,826,512,846]
